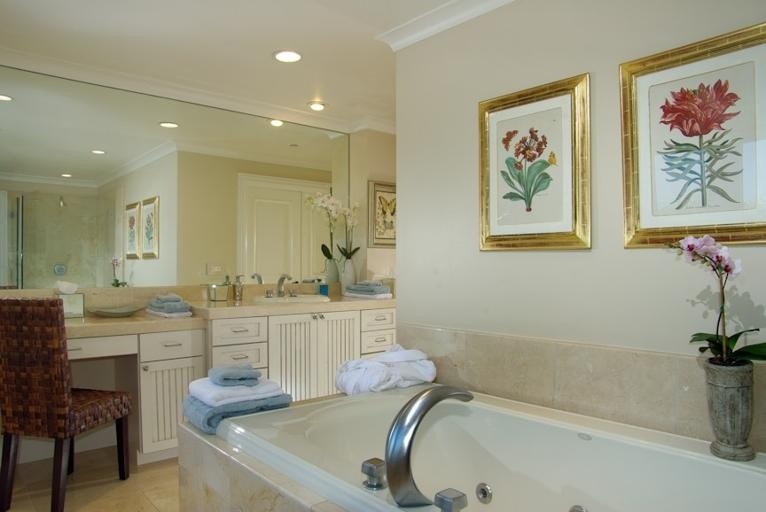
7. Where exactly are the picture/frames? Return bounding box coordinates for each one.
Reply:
[478,72,595,254]
[365,178,396,250]
[617,23,766,250]
[124,201,141,261]
[140,195,160,260]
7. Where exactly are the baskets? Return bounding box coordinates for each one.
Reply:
[291,283,320,295]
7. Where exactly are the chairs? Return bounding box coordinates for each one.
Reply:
[1,295,135,511]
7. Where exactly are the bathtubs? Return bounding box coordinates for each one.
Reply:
[213,378,766,512]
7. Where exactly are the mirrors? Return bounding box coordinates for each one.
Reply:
[56,292,87,319]
[0,64,352,291]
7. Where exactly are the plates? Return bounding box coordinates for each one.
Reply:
[87,305,144,317]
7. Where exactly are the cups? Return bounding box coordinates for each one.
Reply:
[320,285,328,296]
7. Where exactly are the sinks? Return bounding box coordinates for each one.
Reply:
[254,294,331,302]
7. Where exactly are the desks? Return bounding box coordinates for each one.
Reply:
[58,307,209,468]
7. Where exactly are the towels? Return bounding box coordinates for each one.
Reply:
[183,363,295,434]
[145,293,192,319]
[341,280,393,299]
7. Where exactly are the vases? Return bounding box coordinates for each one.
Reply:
[341,260,357,293]
[698,357,757,462]
[324,257,340,285]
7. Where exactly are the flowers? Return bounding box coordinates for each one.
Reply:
[337,201,361,273]
[127,215,136,251]
[657,79,745,214]
[304,190,349,276]
[672,232,766,367]
[144,212,153,250]
[497,126,559,214]
[109,257,127,289]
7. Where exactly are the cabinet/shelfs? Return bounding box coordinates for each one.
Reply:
[273,310,360,403]
[360,307,394,357]
[210,317,268,386]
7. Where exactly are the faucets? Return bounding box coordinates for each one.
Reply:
[384,384,475,508]
[277,273,293,296]
[251,272,263,284]
